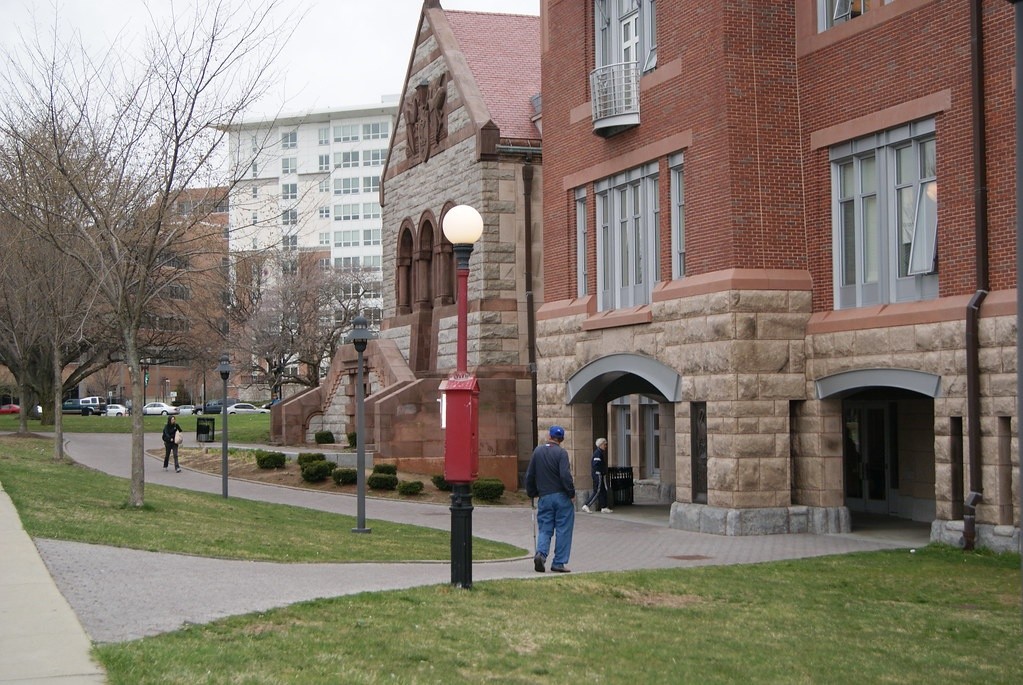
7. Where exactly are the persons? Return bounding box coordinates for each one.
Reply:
[582,438,613,513]
[126,397,132,416]
[162,416,182,472]
[273,393,279,403]
[98,399,106,416]
[525,428,575,572]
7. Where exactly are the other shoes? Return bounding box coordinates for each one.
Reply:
[551,566,572,572]
[163,467,168,471]
[177,468,181,473]
[534,555,545,573]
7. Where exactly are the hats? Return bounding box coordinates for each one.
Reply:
[550,426,564,439]
[596,438,606,447]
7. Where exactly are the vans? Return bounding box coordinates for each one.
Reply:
[81,396,106,404]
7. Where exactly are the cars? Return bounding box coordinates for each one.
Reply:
[0,404,20,415]
[220,402,270,415]
[262,401,274,410]
[177,404,195,415]
[193,398,235,415]
[144,402,180,416]
[101,403,127,417]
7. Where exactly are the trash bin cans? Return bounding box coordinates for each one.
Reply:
[82,406,89,416]
[608,466,634,506]
[196,417,215,442]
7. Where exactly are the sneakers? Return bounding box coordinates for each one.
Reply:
[582,505,593,514]
[600,507,613,513]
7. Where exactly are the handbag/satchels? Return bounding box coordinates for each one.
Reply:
[174,430,183,444]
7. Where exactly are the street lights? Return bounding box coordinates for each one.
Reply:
[437,202,486,594]
[216,354,234,501]
[140,364,150,406]
[347,316,375,535]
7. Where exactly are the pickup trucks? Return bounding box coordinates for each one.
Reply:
[61,398,108,417]
